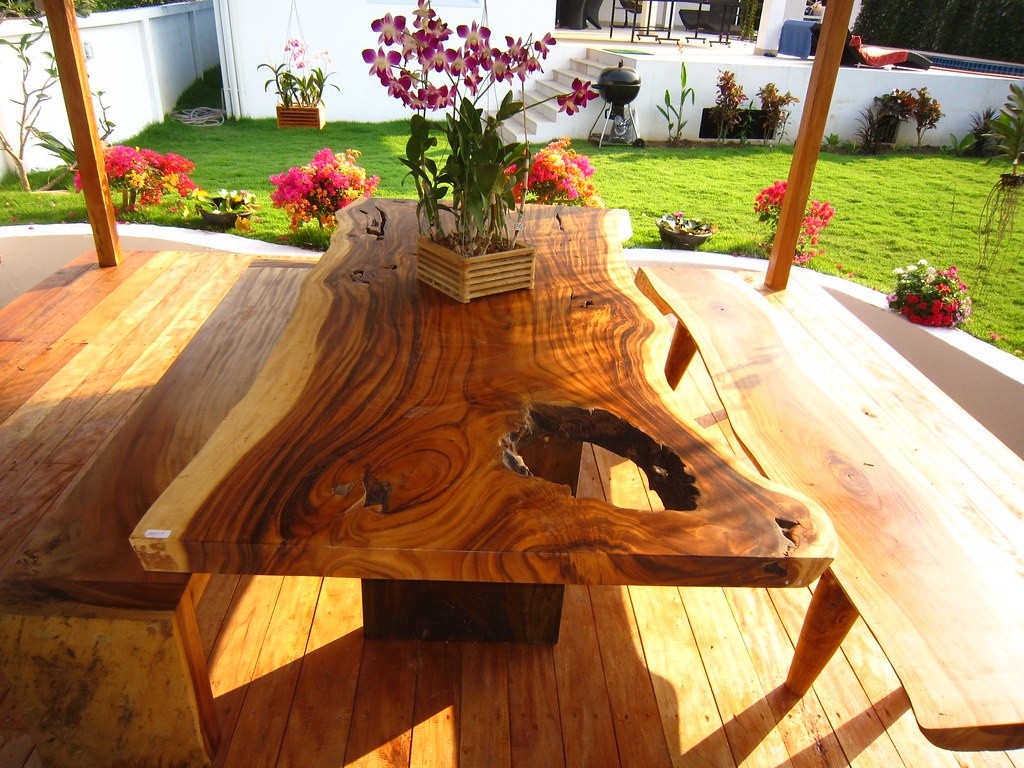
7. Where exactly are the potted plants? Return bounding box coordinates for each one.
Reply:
[981,83,1024,188]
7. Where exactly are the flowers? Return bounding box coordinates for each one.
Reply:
[753,180,834,261]
[182,187,265,232]
[504,137,605,211]
[268,146,381,232]
[656,210,719,236]
[362,0,599,258]
[885,258,974,330]
[257,40,341,108]
[70,146,203,211]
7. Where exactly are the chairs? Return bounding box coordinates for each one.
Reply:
[678,9,743,35]
[809,23,933,71]
[610,0,642,28]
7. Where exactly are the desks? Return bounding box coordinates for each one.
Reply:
[132,195,836,647]
[610,0,742,47]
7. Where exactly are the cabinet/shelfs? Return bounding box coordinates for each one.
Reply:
[803,6,825,24]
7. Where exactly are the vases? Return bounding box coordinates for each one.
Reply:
[414,232,537,303]
[195,197,254,228]
[274,103,327,131]
[659,227,713,249]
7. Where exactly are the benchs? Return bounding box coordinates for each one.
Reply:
[632,262,1024,755]
[0,259,317,768]
